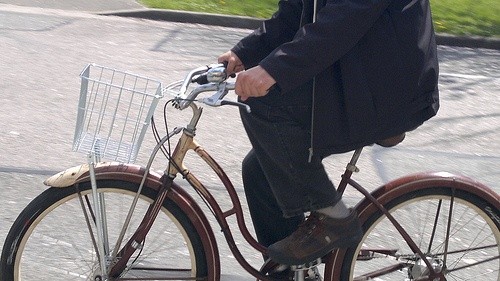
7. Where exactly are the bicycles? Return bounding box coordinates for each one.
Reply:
[0,60,500,281]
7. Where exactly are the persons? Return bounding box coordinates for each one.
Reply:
[219,0,440,281]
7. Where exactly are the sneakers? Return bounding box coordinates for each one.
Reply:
[267,208,362,264]
